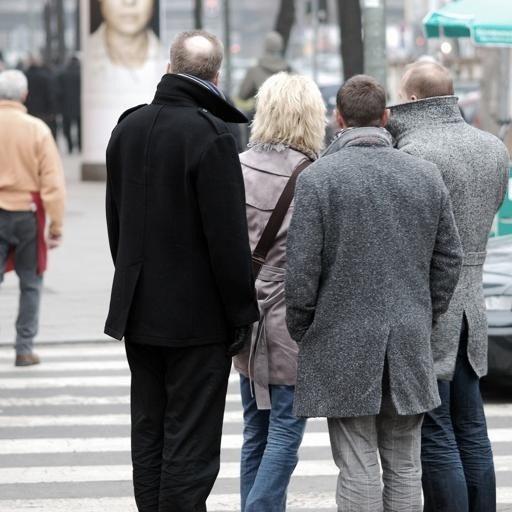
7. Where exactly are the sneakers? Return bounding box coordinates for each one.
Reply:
[15,353,40,367]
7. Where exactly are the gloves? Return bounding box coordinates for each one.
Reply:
[228,325,253,357]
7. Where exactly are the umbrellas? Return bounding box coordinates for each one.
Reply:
[421,0,512,46]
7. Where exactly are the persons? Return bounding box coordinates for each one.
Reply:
[1,49,84,155]
[102,27,259,510]
[238,31,296,101]
[286,72,465,512]
[1,69,66,368]
[89,2,158,149]
[233,70,329,511]
[381,56,509,512]
